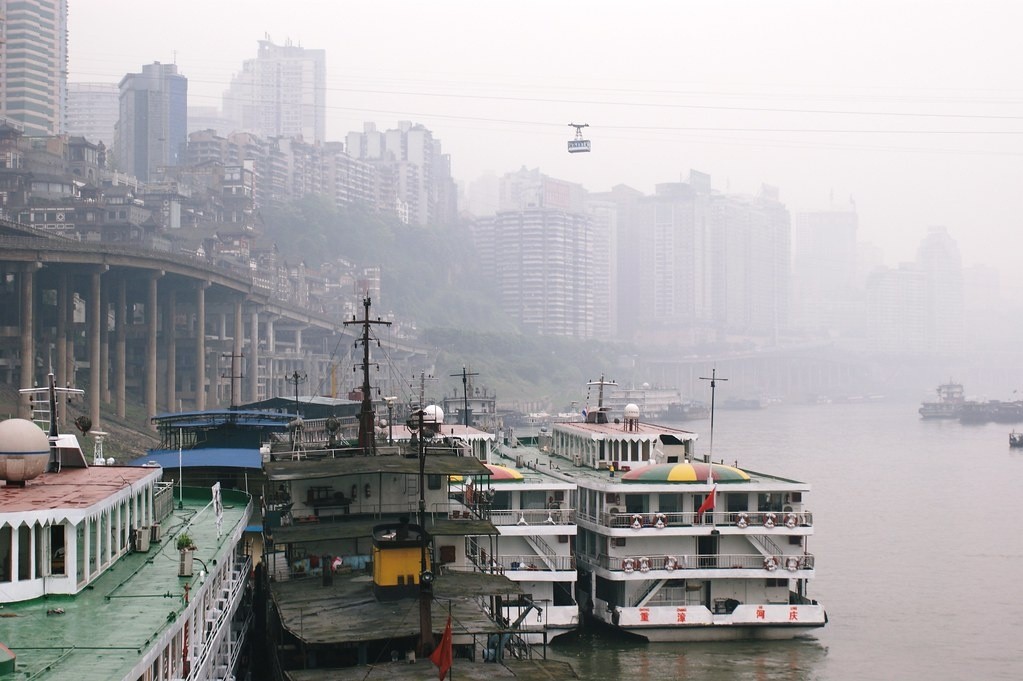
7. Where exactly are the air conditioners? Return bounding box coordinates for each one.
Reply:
[608,506,626,517]
[782,504,801,512]
[573,456,582,467]
[549,503,567,516]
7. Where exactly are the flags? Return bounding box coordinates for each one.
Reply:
[698,487,715,513]
[582,406,588,417]
[431,618,451,681]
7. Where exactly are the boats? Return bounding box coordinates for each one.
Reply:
[496,402,827,640]
[129,372,361,488]
[372,397,580,647]
[0,346,262,681]
[917,382,1023,424]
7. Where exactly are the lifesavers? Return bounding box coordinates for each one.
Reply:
[763,557,779,570]
[783,513,797,528]
[630,514,644,528]
[621,558,636,573]
[736,513,749,527]
[653,513,667,527]
[663,556,677,569]
[638,557,651,571]
[786,556,799,571]
[763,512,777,528]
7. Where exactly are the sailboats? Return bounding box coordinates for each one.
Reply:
[258,292,580,680]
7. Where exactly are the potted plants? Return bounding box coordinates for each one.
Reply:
[151,526,161,543]
[135,528,150,552]
[176,532,196,553]
